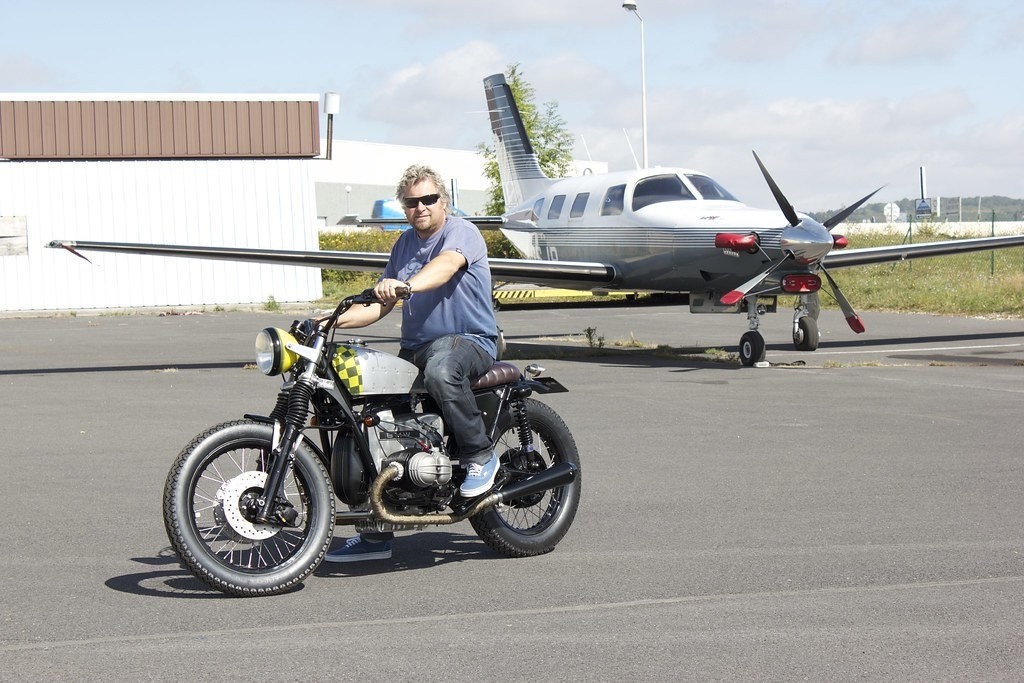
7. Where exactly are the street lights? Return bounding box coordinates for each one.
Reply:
[622,1,650,169]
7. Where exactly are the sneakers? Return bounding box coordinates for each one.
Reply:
[460,452,500,497]
[324,536,392,562]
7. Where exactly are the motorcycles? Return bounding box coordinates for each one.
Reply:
[162,287,582,597]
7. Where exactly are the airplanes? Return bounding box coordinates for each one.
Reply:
[43,74,1024,368]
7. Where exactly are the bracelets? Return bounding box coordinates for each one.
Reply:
[402,281,410,286]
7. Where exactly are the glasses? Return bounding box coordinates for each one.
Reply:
[403,192,440,208]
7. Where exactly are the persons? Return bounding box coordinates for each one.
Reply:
[295,164,501,562]
